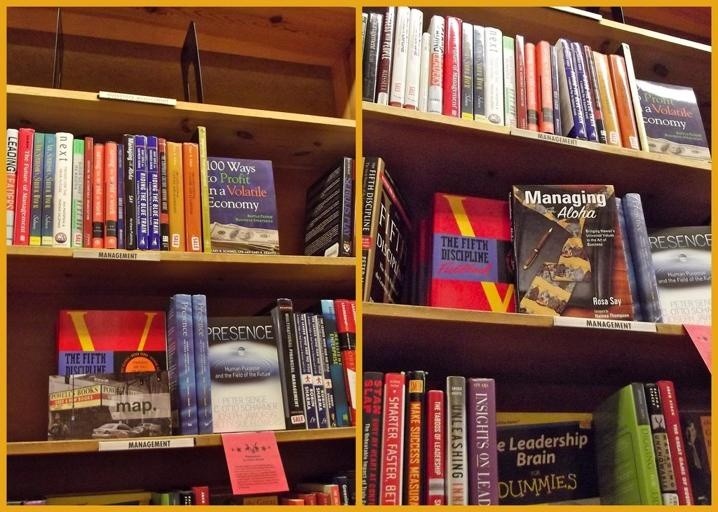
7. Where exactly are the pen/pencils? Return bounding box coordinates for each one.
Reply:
[522,227,553,270]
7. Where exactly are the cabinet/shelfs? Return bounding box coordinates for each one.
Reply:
[359,0,718,511]
[2,0,357,511]
